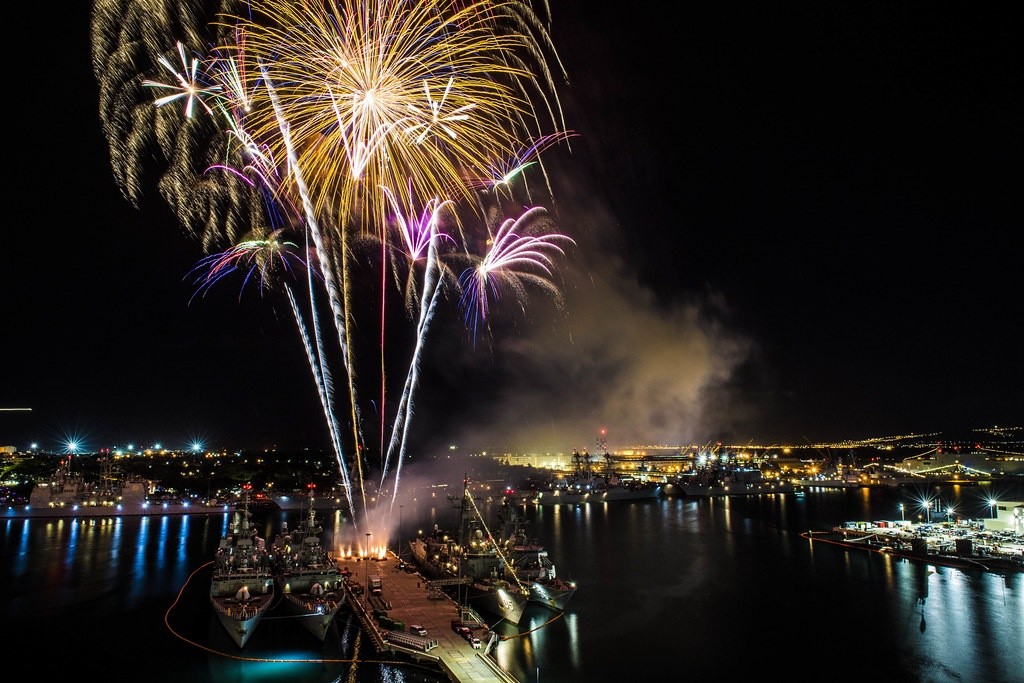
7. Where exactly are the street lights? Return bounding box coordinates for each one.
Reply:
[365,533,371,612]
[398,505,405,558]
[947,508,953,522]
[900,504,904,521]
[924,501,932,523]
[990,499,995,519]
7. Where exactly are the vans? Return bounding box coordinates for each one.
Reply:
[409,624,428,638]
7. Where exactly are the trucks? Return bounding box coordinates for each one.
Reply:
[367,574,383,594]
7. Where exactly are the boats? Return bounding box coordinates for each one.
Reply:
[549,440,799,504]
[408,466,531,628]
[209,480,275,650]
[268,481,346,644]
[484,495,578,615]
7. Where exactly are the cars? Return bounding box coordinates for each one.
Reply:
[456,627,474,643]
[471,637,482,650]
